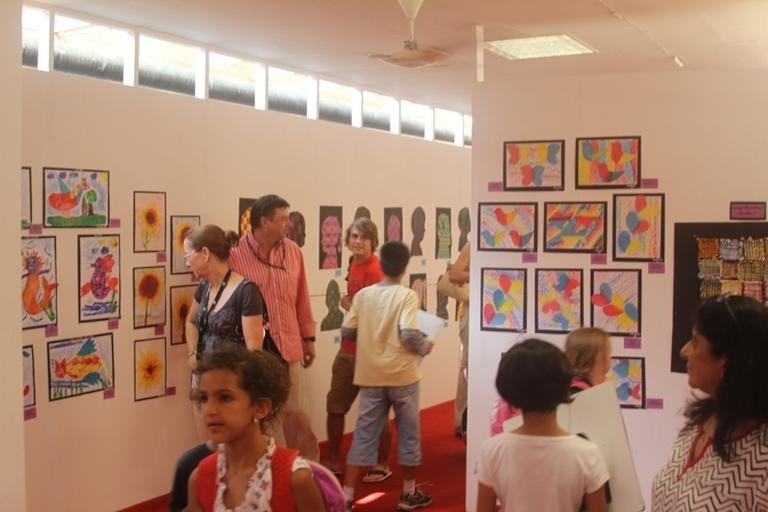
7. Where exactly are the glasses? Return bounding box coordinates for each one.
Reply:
[182,250,197,261]
[716,290,742,326]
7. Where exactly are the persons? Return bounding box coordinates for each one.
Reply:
[565,328,612,394]
[342,242,434,510]
[322,219,391,482]
[184,225,287,448]
[652,295,768,512]
[448,231,470,442]
[477,339,612,512]
[227,195,315,410]
[168,345,324,511]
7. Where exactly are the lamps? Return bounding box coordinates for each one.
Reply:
[369,1,447,71]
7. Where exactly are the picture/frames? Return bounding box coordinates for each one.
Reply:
[728,200,766,220]
[476,201,537,253]
[41,165,110,229]
[574,135,640,190]
[76,233,121,324]
[606,355,647,409]
[588,267,641,339]
[534,268,583,336]
[169,284,200,346]
[22,344,36,409]
[132,265,166,330]
[46,332,114,402]
[611,192,664,263]
[542,200,606,254]
[134,336,166,401]
[169,214,201,275]
[133,190,166,253]
[22,234,58,330]
[478,267,527,334]
[21,166,31,230]
[503,139,564,193]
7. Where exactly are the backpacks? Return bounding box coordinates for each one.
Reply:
[491,381,590,436]
[195,450,345,512]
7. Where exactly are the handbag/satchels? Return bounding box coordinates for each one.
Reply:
[236,279,290,367]
[437,263,469,304]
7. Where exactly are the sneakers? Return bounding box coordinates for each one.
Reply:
[396,486,433,511]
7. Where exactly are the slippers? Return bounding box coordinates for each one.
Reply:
[323,463,343,475]
[362,469,393,483]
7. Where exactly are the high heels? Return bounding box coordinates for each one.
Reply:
[454,428,464,440]
